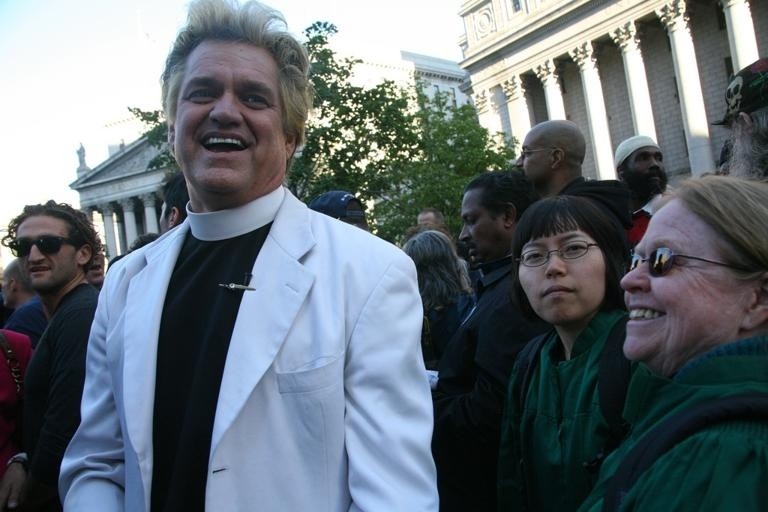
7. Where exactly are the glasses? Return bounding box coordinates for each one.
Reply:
[520,147,557,160]
[6,236,84,256]
[628,247,754,279]
[513,239,613,268]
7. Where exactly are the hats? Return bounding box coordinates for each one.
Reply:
[708,56,767,125]
[612,135,663,180]
[308,190,366,219]
